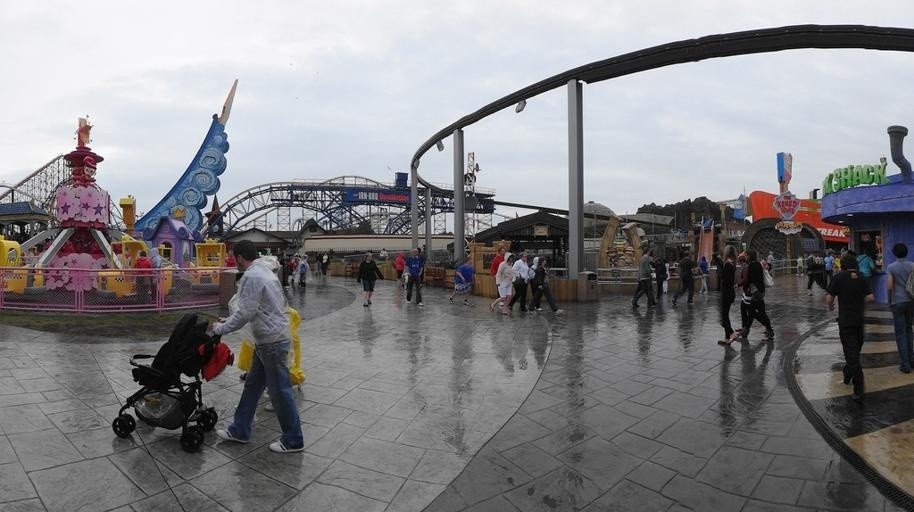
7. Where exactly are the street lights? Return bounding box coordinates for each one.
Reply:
[587,199,598,270]
[297,219,302,255]
[267,224,272,249]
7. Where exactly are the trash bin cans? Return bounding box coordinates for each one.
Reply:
[220,270,244,308]
[652,280,664,304]
[278,265,288,286]
[577,271,598,303]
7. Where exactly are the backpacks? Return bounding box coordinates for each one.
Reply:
[200,340,234,382]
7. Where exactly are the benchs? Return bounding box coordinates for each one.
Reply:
[423,266,446,288]
[343,261,361,277]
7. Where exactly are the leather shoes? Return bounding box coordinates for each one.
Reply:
[632,301,641,307]
[718,329,774,346]
[647,303,657,308]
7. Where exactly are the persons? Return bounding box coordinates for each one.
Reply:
[134,251,152,303]
[490,248,506,305]
[380,251,383,256]
[632,247,775,345]
[827,253,875,401]
[226,254,236,267]
[508,251,531,313]
[415,247,425,288]
[489,252,516,316]
[796,246,876,295]
[4,230,52,257]
[395,252,405,282]
[150,248,160,303]
[281,249,331,288]
[448,256,474,306]
[380,247,388,259]
[209,238,305,453]
[885,243,914,373]
[530,256,544,311]
[404,248,424,306]
[528,257,564,315]
[356,251,386,307]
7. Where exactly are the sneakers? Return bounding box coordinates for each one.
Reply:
[490,304,565,315]
[464,300,474,305]
[417,302,424,306]
[899,366,910,373]
[844,365,863,394]
[449,298,455,304]
[363,299,372,306]
[687,300,695,305]
[270,438,306,453]
[216,428,249,442]
[406,298,412,303]
[672,299,678,305]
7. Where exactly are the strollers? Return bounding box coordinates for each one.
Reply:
[108,307,233,456]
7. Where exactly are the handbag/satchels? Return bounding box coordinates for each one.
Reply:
[905,269,914,298]
